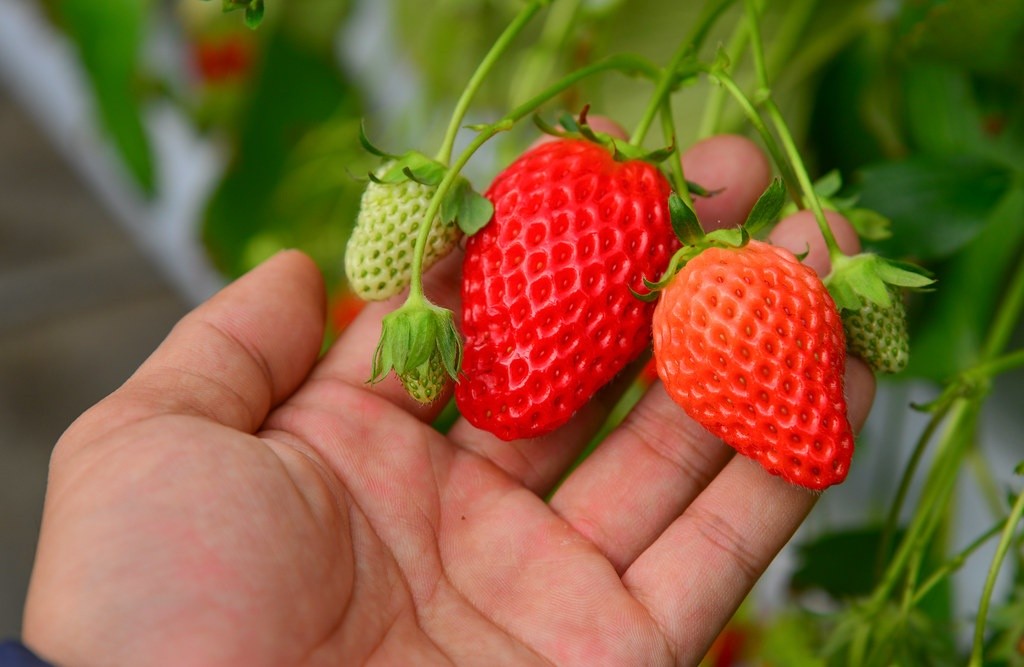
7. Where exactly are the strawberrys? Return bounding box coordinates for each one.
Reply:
[696,619,751,667]
[344,121,933,495]
[191,33,254,80]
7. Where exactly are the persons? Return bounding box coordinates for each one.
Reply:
[0,112,876,667]
